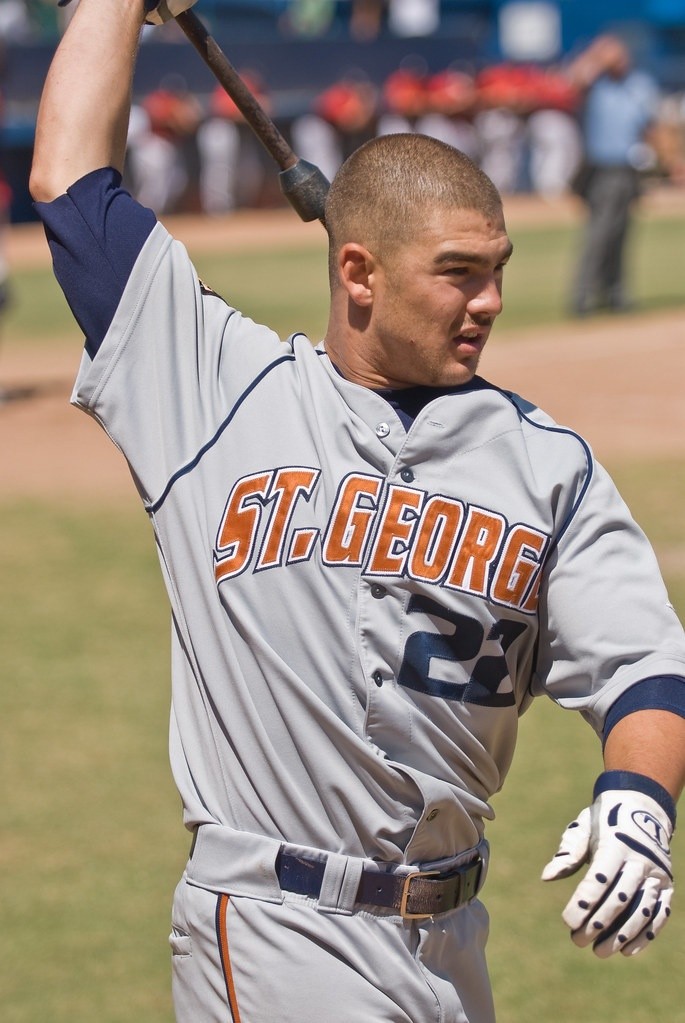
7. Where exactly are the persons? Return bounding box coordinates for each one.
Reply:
[565,31,681,314]
[27,0,685,1023]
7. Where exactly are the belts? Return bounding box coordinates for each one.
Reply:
[189,824,483,920]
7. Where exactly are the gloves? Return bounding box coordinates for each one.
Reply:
[145,1,198,25]
[539,769,676,960]
